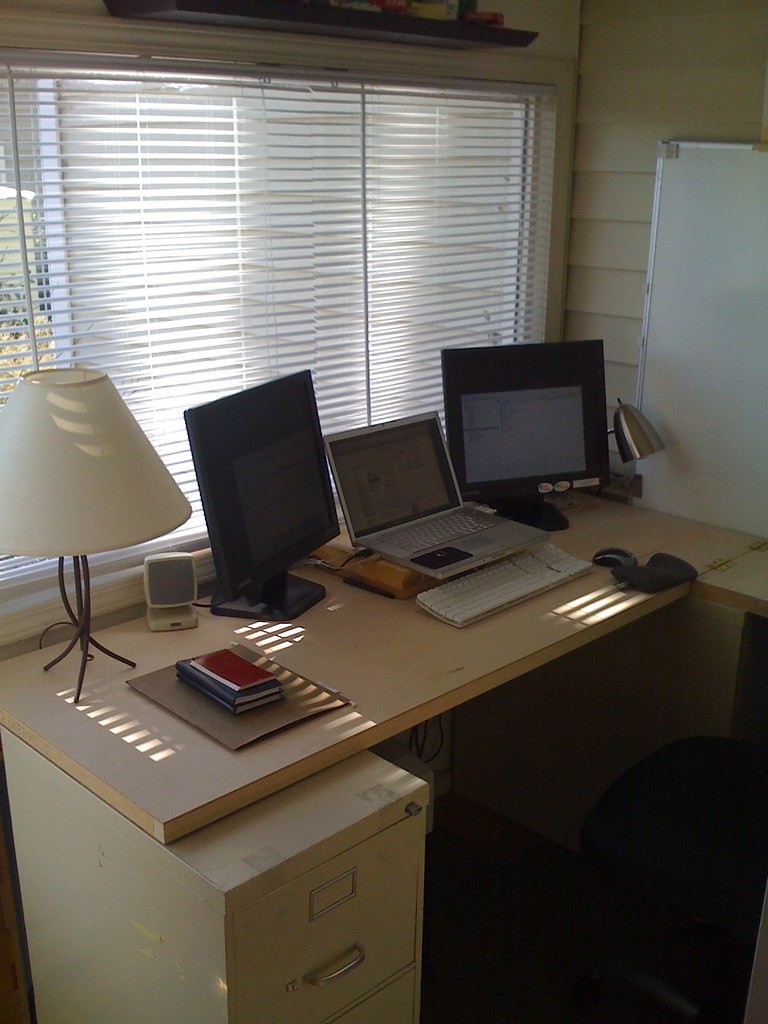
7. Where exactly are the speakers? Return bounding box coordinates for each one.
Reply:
[144,551,198,631]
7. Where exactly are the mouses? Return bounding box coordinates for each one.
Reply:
[591,547,638,569]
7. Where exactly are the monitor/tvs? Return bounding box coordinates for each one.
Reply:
[441,339,610,531]
[184,369,341,620]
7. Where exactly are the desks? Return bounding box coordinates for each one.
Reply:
[0,488,768,1024]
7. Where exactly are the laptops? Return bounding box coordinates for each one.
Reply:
[322,411,553,580]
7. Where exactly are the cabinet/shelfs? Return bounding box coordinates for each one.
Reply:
[0,723,426,1024]
[102,0,539,50]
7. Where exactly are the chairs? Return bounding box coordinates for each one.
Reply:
[568,735,768,1023]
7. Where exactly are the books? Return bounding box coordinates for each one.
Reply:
[175,649,285,714]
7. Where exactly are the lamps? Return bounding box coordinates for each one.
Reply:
[607,396,665,464]
[0,368,192,706]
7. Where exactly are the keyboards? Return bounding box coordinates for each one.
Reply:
[416,542,593,627]
